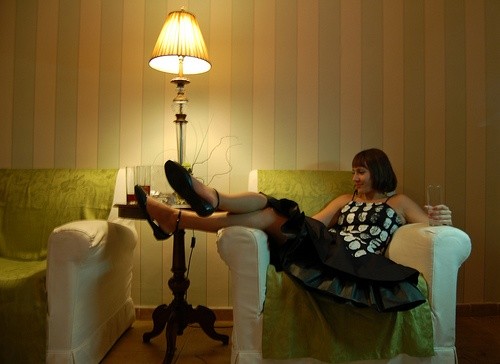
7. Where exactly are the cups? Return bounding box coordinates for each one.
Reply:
[427,184,442,226]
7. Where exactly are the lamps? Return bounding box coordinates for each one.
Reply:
[148,8,213,210]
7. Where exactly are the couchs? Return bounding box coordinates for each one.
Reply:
[217,169,471,364]
[0,167,136,364]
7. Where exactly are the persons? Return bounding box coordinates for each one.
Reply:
[134,148,452,311]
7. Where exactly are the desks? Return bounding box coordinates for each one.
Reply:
[120,205,230,364]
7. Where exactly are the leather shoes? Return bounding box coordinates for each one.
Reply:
[134,184,182,240]
[164,159,220,217]
